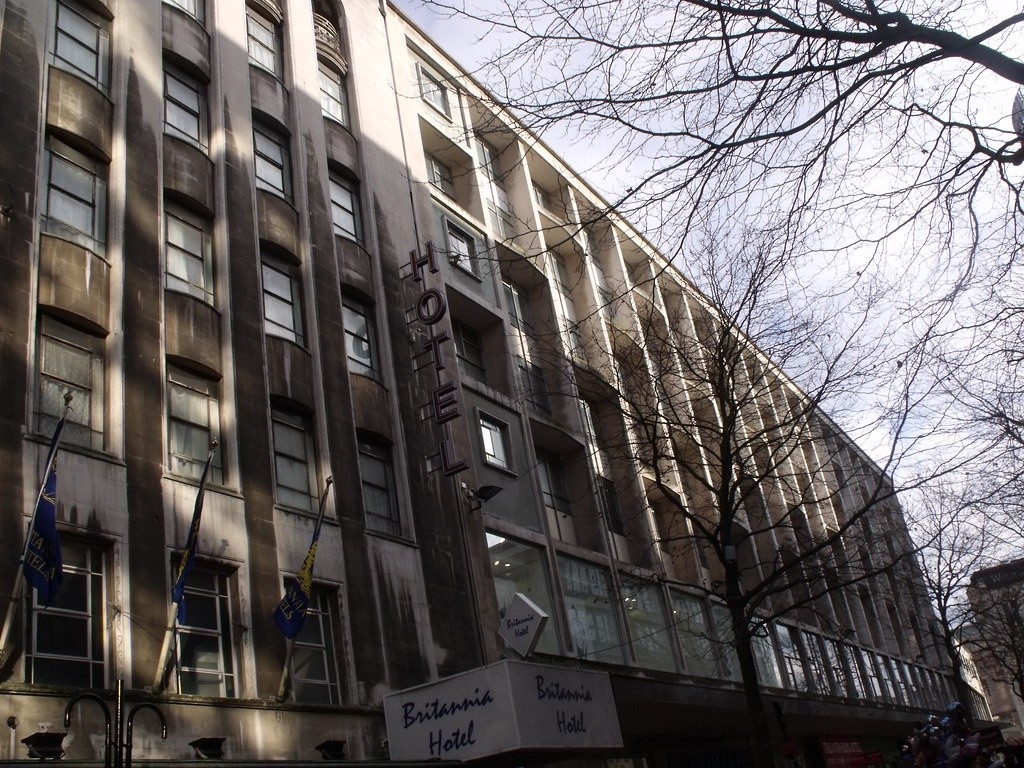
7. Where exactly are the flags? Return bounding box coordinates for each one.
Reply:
[166,457,213,657]
[13,412,63,598]
[275,493,326,639]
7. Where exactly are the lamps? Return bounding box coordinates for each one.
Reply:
[21,732,67,761]
[469,486,503,511]
[189,738,226,760]
[315,740,346,760]
